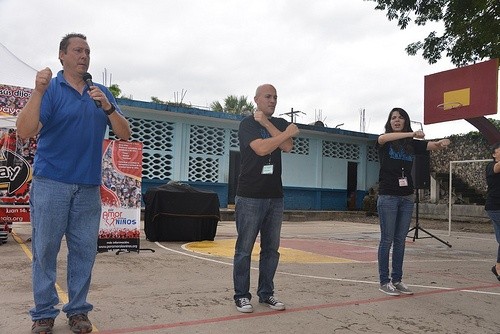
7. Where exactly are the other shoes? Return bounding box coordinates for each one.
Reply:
[379,283,400,295]
[259,296,285,310]
[491,265,500,281]
[235,298,254,312]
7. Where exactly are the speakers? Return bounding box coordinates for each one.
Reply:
[411,152,432,188]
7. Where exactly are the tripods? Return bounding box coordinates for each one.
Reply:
[407,188,453,249]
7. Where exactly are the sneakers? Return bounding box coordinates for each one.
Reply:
[68,314,92,334]
[392,282,412,294]
[32,318,54,334]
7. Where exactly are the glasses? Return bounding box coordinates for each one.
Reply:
[495,143,500,149]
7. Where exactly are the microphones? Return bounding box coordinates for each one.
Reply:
[82,72,102,107]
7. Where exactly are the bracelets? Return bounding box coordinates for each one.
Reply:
[103,102,116,115]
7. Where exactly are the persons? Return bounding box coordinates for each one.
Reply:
[102,143,141,209]
[484,143,500,282]
[376,107,451,296]
[232,83,300,313]
[15,33,131,334]
[0,84,38,166]
